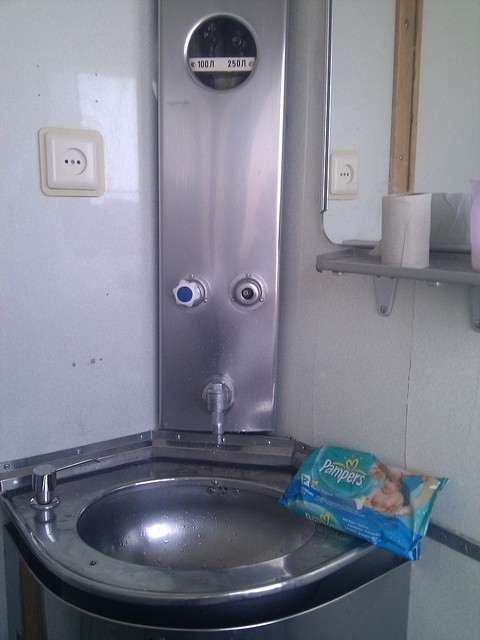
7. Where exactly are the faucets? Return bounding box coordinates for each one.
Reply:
[202,382,233,444]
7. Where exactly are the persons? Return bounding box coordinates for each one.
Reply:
[370,457,434,515]
[363,469,405,514]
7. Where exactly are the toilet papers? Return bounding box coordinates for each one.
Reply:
[369,191,431,269]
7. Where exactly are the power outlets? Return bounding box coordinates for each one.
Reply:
[38,129,104,199]
[328,148,363,199]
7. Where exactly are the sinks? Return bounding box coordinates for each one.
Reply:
[8,457,412,632]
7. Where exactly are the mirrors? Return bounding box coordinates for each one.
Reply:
[321,1,480,251]
